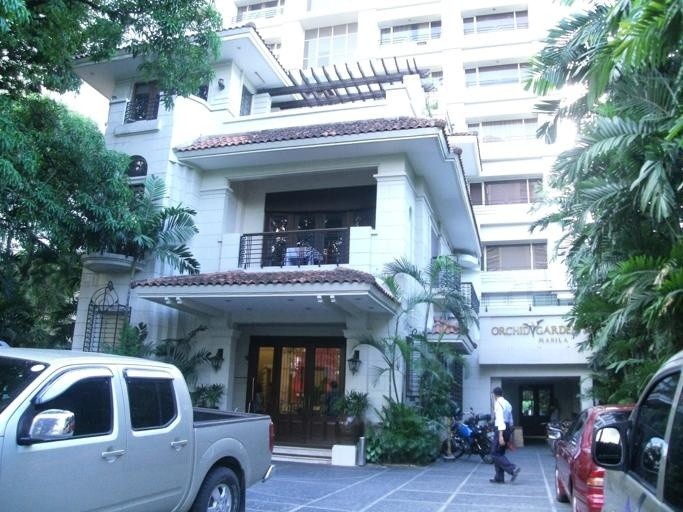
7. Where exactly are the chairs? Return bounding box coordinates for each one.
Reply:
[267,236,343,268]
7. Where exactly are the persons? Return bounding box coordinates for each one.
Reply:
[548,403,560,421]
[321,380,341,441]
[489,386,520,483]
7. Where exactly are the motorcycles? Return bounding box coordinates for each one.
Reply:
[441,407,495,464]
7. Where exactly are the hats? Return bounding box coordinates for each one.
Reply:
[491,387,502,394]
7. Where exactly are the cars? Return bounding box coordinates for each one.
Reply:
[545,349,683,512]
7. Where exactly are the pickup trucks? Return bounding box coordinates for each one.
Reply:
[0,347,276,512]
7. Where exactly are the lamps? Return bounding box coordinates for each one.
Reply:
[212,349,224,371]
[346,348,362,375]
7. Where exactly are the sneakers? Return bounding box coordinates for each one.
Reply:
[489,478,504,484]
[510,466,521,483]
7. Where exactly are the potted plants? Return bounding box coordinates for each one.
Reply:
[329,389,369,446]
[195,384,224,409]
